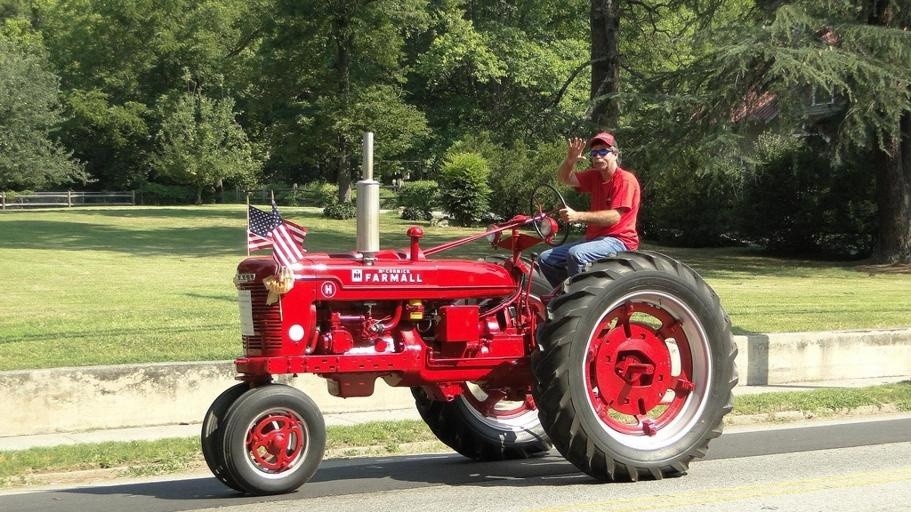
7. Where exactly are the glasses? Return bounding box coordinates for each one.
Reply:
[591,148,613,156]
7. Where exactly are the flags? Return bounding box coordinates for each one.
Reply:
[246,194,308,253]
[271,190,308,277]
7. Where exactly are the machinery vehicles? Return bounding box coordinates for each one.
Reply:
[201,131,740,497]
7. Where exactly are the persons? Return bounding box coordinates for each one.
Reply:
[537,130,643,293]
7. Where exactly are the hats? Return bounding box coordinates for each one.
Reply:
[589,132,617,146]
